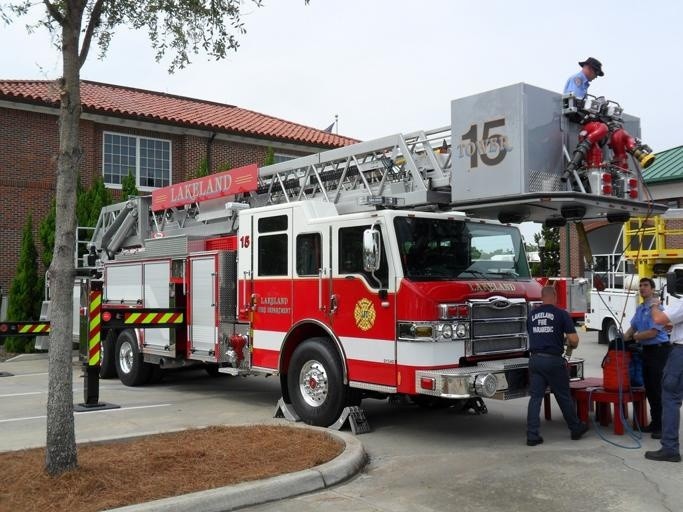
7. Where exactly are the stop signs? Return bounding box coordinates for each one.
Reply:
[34,82,668,427]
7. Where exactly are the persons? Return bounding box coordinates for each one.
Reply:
[622,270,683,462]
[523,286,589,447]
[562,57,604,100]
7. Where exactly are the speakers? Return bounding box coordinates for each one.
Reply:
[640,408,662,439]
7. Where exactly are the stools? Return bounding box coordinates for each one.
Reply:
[642,341,670,350]
[531,351,561,358]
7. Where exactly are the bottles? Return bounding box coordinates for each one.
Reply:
[579,57,605,76]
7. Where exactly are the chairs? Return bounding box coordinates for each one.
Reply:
[570,421,588,441]
[644,447,681,462]
[526,437,544,446]
